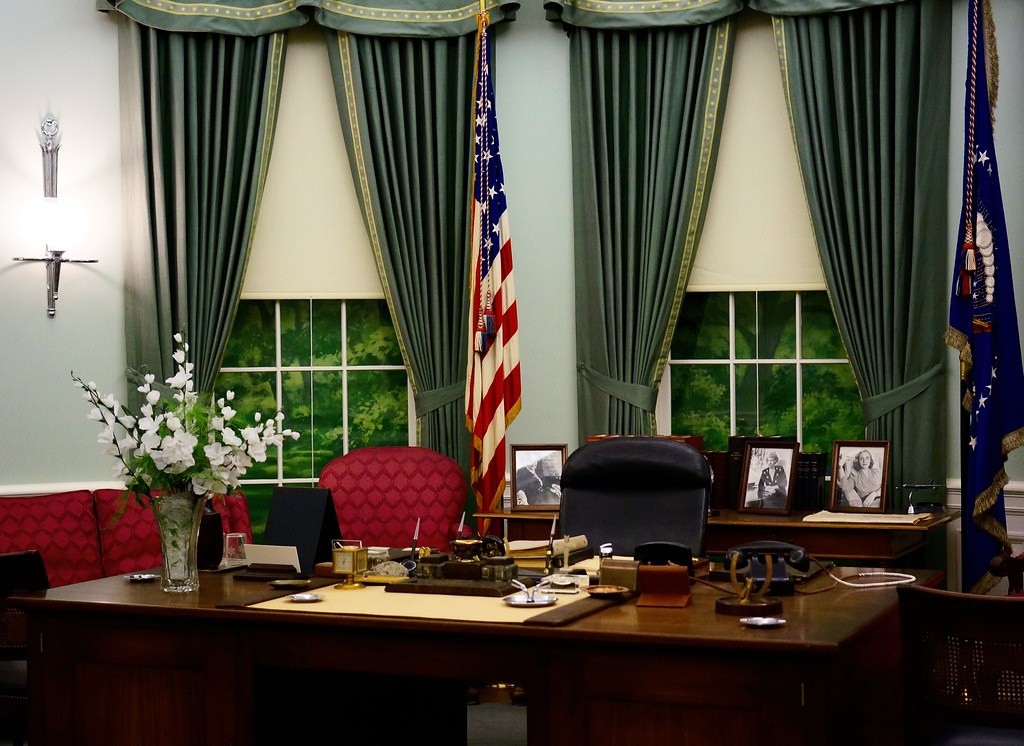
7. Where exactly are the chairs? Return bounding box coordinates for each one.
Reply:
[319,447,467,556]
[896,584,1024,746]
[559,439,711,558]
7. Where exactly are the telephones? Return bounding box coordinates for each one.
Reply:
[722,540,811,596]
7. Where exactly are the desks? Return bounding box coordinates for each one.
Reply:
[471,508,962,591]
[5,565,912,746]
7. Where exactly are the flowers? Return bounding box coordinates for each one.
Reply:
[67,330,299,499]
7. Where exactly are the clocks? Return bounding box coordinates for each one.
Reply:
[329,540,369,590]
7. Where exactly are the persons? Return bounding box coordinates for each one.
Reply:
[465,10,523,538]
[837,449,883,507]
[942,0,1024,595]
[757,452,787,509]
[516,454,559,505]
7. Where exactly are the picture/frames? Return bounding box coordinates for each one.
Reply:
[510,442,569,512]
[737,437,799,518]
[831,441,890,513]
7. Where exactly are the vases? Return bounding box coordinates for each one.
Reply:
[149,492,203,592]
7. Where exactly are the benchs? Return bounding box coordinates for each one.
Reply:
[1,491,255,705]
